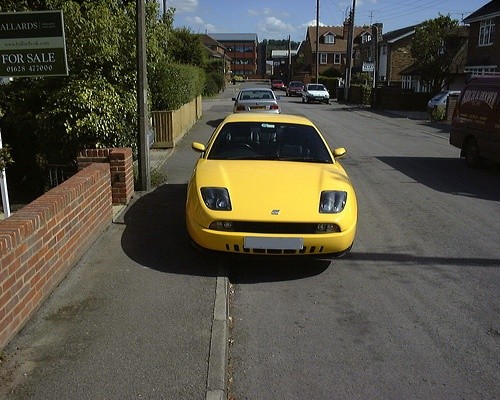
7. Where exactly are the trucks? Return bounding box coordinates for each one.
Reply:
[449,72,500,167]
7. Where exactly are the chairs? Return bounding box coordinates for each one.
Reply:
[263,94,269,98]
[243,95,250,99]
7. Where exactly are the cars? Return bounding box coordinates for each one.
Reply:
[427,89,461,112]
[271,78,284,91]
[301,83,330,103]
[232,87,281,114]
[286,80,305,96]
[185,113,357,258]
